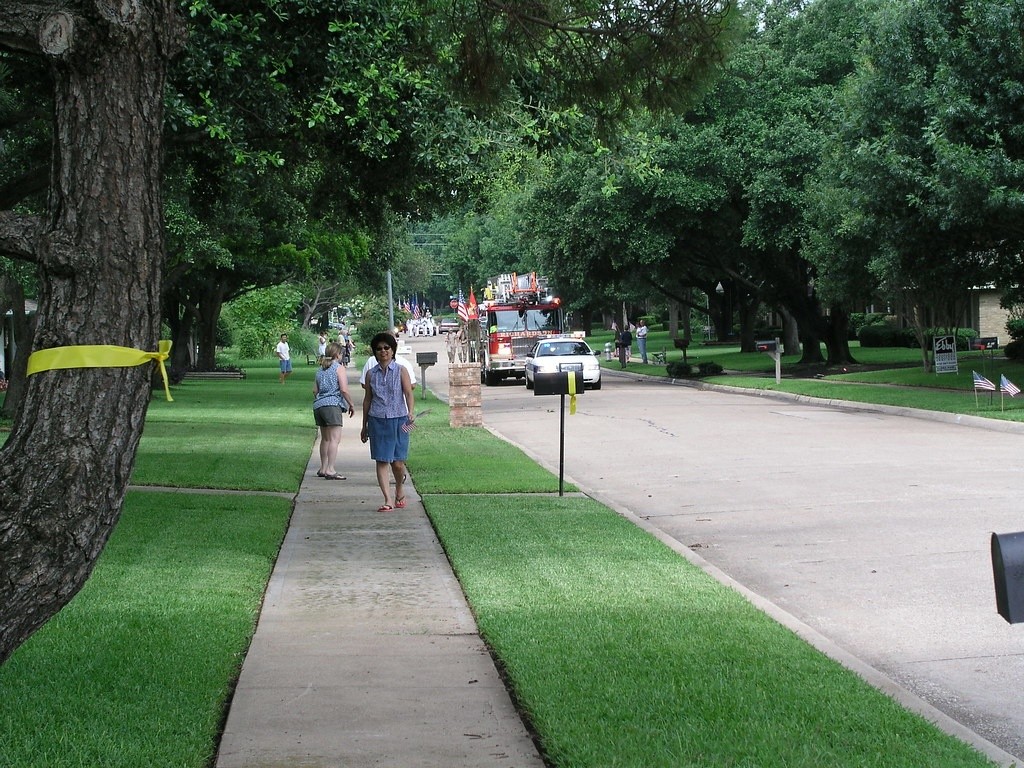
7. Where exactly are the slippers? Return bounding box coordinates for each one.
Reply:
[326,472,347,480]
[318,471,325,477]
[395,496,405,507]
[378,505,395,512]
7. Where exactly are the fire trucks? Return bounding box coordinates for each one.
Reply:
[469,272,563,387]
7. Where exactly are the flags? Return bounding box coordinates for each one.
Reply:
[1000,375,1021,396]
[973,370,995,390]
[458,290,468,322]
[468,291,482,317]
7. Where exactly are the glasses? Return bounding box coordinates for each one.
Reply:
[375,346,391,351]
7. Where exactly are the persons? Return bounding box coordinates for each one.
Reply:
[612,325,633,361]
[312,342,354,480]
[635,318,648,364]
[328,329,353,365]
[319,330,327,366]
[276,333,292,381]
[407,300,433,319]
[359,332,416,513]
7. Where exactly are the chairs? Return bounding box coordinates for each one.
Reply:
[306,354,316,365]
[652,346,667,365]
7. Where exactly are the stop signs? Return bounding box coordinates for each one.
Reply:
[450,300,458,308]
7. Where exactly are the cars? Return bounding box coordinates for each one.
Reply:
[524,333,602,391]
[439,318,460,334]
[310,320,343,330]
